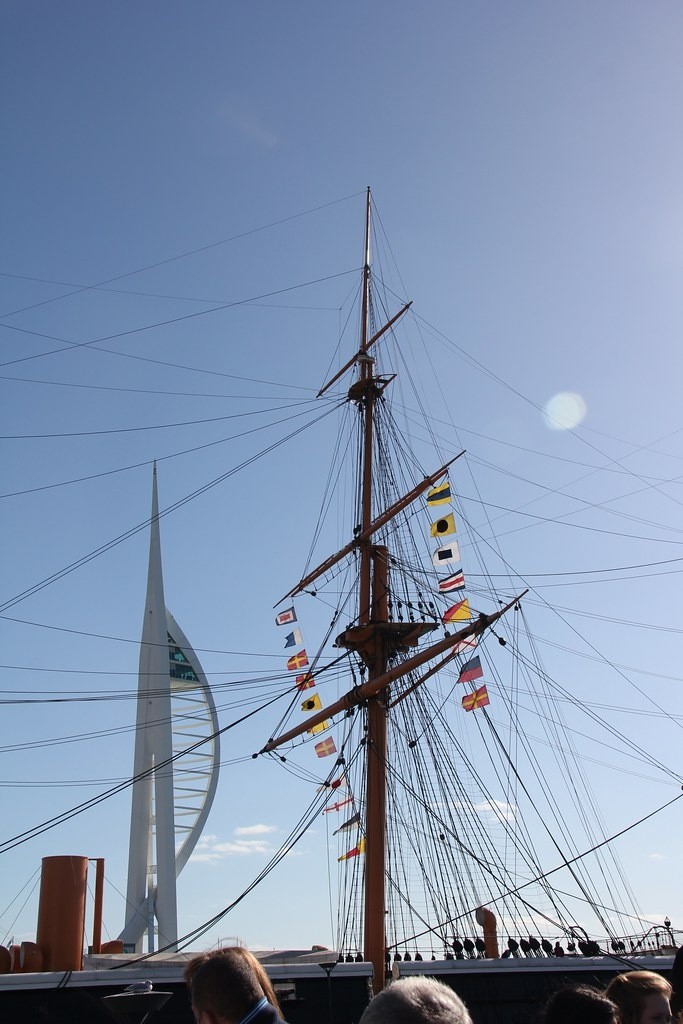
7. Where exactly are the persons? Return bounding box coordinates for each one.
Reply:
[184,947,289,1024]
[540,982,621,1024]
[602,970,672,1024]
[359,974,474,1024]
[670,945,683,1024]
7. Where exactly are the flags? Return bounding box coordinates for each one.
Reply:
[276,606,367,862]
[426,481,490,712]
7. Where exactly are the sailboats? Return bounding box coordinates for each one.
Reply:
[0,181,683,1024]
